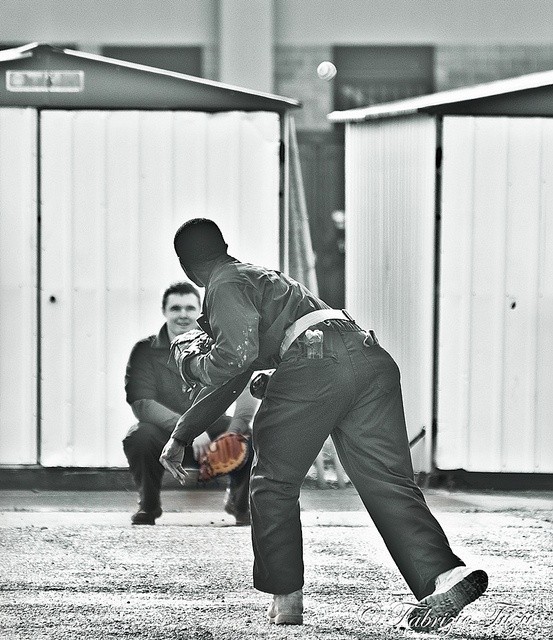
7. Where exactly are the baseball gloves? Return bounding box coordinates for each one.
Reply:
[199,433,249,481]
[169,330,214,384]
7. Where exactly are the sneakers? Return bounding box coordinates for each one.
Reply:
[408,569,490,630]
[266,592,307,625]
[133,505,161,522]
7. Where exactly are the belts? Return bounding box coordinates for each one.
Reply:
[278,307,354,357]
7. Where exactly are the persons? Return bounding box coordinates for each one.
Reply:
[174,218,488,631]
[122,282,254,526]
